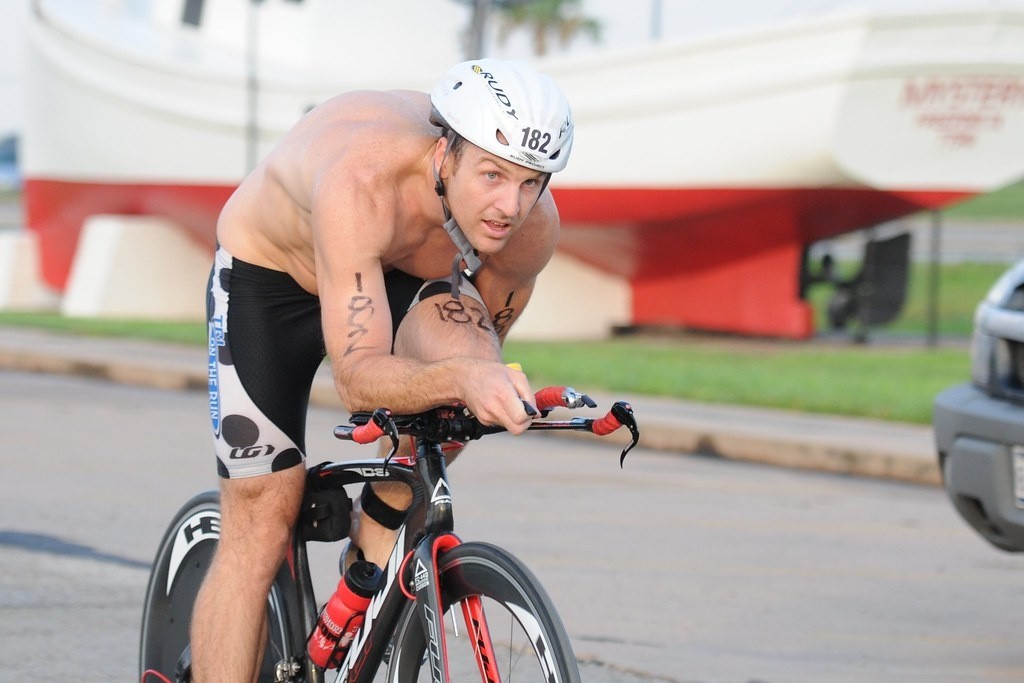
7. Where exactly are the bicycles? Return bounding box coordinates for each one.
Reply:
[136,386,640,683]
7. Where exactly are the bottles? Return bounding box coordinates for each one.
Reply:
[307,562,385,668]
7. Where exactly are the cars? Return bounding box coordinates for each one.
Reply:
[930,261,1024,554]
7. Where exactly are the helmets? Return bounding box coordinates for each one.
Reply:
[426,58,572,174]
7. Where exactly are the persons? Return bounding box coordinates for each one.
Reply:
[190,59,574,683]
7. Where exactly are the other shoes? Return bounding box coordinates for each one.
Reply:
[342,544,390,622]
